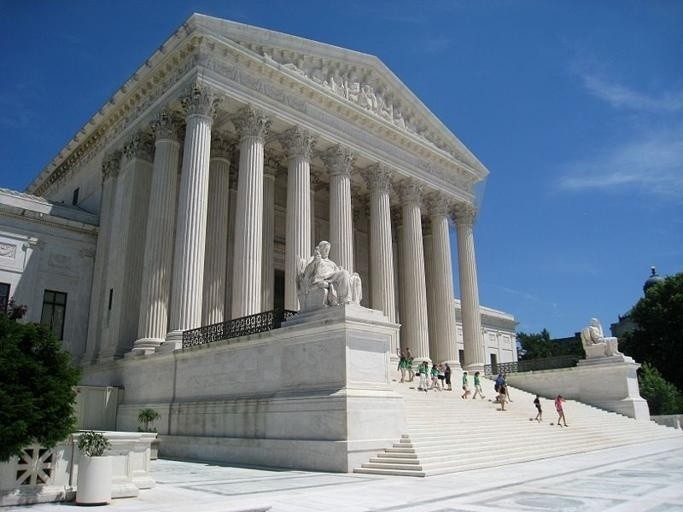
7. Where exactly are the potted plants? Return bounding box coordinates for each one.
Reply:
[74,429,112,505]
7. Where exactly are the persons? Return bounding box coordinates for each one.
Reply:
[554,394,568,427]
[501,373,513,403]
[499,383,508,411]
[460,372,470,399]
[584,318,623,356]
[300,240,362,306]
[261,51,419,136]
[495,371,504,401]
[396,346,453,392]
[532,394,543,421]
[471,371,485,399]
